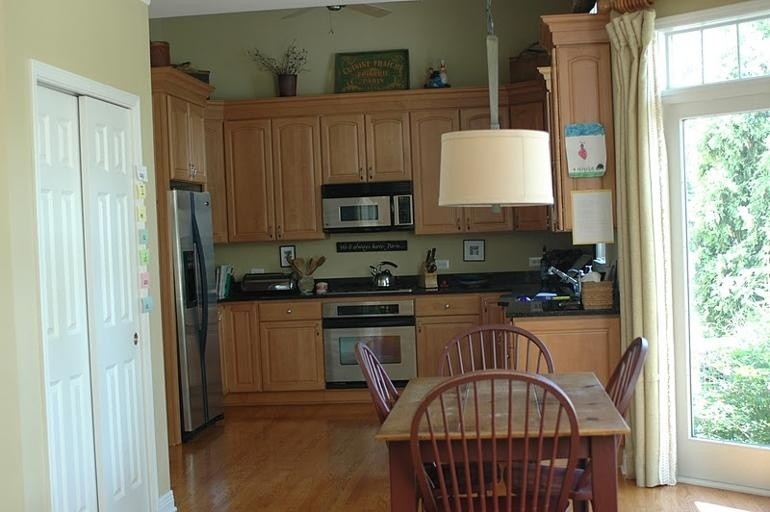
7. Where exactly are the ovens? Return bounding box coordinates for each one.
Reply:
[322,317,418,388]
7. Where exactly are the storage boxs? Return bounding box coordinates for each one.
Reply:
[509,52,550,83]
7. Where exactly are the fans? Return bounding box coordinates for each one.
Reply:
[281,4,392,20]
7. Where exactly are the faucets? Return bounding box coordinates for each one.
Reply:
[548,264,581,297]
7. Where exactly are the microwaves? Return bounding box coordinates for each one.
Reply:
[321,180,415,233]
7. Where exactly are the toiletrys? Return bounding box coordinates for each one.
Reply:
[595,241,606,263]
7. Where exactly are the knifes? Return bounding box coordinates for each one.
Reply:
[425,248,437,273]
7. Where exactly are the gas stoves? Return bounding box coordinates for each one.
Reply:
[320,290,417,319]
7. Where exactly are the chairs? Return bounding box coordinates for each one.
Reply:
[353,340,501,512]
[502,336,649,512]
[409,367,579,512]
[438,322,554,375]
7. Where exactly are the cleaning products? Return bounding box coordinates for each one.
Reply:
[579,265,603,282]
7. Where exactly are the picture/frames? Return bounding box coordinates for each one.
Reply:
[463,239,485,262]
[279,245,296,268]
[334,48,410,93]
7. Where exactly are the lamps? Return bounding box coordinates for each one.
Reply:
[437,0,555,208]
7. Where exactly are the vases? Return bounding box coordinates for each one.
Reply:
[278,74,297,97]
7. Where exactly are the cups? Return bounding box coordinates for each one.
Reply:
[298,276,315,296]
[316,281,328,296]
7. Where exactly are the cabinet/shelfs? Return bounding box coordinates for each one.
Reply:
[259,300,326,392]
[204,118,228,244]
[550,43,617,234]
[508,315,622,388]
[152,93,207,185]
[409,104,515,236]
[218,302,263,394]
[415,293,483,377]
[320,110,412,185]
[510,101,549,232]
[483,292,508,369]
[223,115,331,243]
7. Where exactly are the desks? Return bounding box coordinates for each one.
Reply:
[374,370,631,512]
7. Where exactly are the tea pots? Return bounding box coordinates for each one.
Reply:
[368,261,398,288]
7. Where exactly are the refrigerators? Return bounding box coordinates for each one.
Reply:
[168,189,226,444]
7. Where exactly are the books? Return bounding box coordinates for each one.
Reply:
[216,264,231,299]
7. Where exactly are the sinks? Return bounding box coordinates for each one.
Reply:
[516,293,572,305]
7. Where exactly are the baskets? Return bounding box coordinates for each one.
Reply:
[580,280,614,310]
